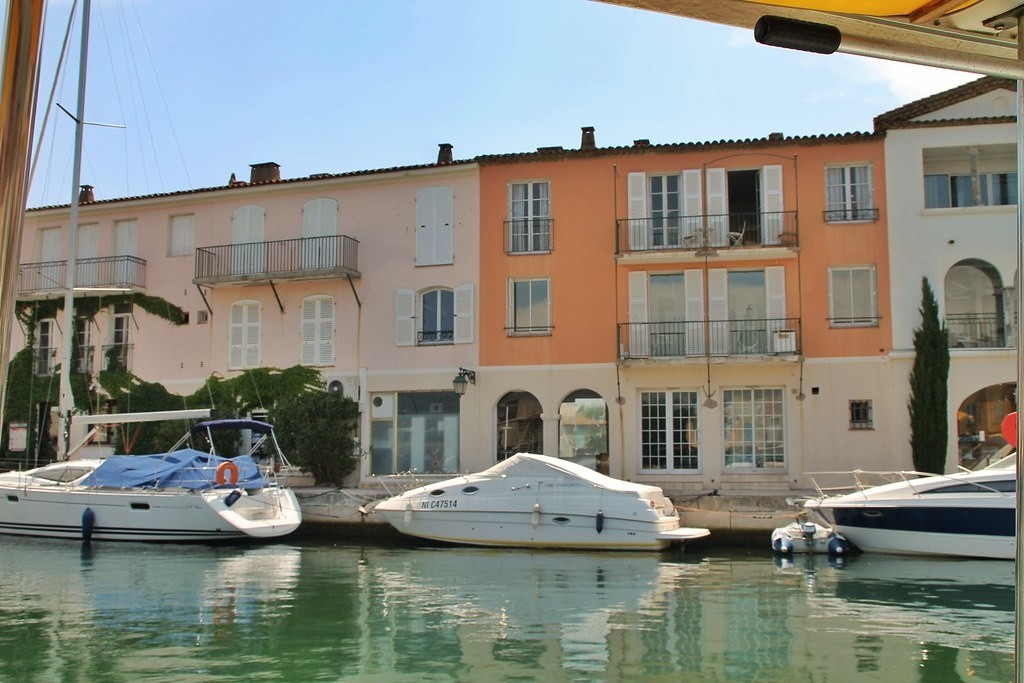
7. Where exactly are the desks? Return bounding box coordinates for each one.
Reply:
[696,227,715,246]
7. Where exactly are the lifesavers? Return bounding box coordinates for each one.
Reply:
[216,461,238,484]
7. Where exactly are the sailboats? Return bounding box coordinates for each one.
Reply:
[1,0,303,541]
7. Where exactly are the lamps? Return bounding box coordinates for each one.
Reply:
[453,367,475,397]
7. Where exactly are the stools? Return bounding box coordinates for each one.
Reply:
[682,235,697,248]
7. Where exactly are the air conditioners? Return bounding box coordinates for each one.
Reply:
[326,375,358,402]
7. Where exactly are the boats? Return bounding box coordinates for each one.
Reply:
[375,452,711,550]
[772,520,849,555]
[792,412,1017,560]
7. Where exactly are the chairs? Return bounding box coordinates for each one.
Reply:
[727,221,746,250]
